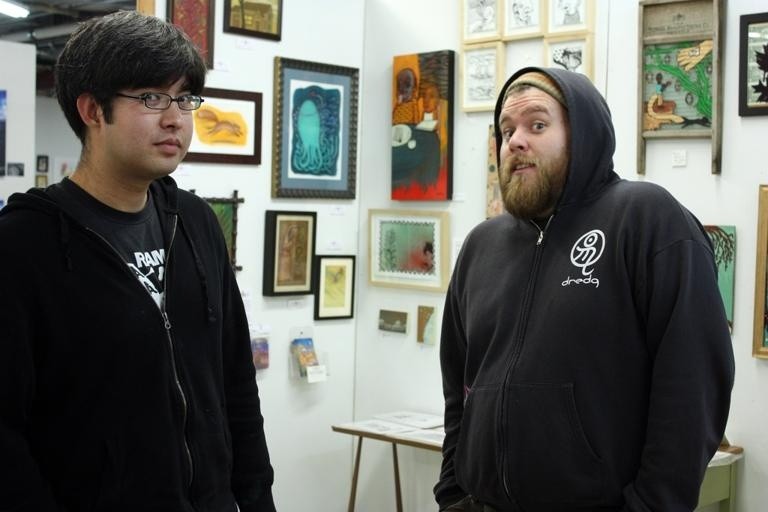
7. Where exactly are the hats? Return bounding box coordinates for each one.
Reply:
[502,71,565,104]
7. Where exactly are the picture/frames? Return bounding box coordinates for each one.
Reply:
[270,54,360,201]
[458,0,604,118]
[366,206,452,295]
[634,0,726,181]
[188,187,246,278]
[312,254,356,322]
[261,209,318,298]
[165,0,216,72]
[221,0,286,44]
[36,155,48,172]
[735,9,768,119]
[179,85,262,166]
[700,223,739,340]
[36,175,48,189]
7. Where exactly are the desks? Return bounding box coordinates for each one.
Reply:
[330,412,743,512]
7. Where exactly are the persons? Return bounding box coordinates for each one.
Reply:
[433,66,736,512]
[1,12,276,512]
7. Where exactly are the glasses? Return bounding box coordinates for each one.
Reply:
[117,91,203,111]
